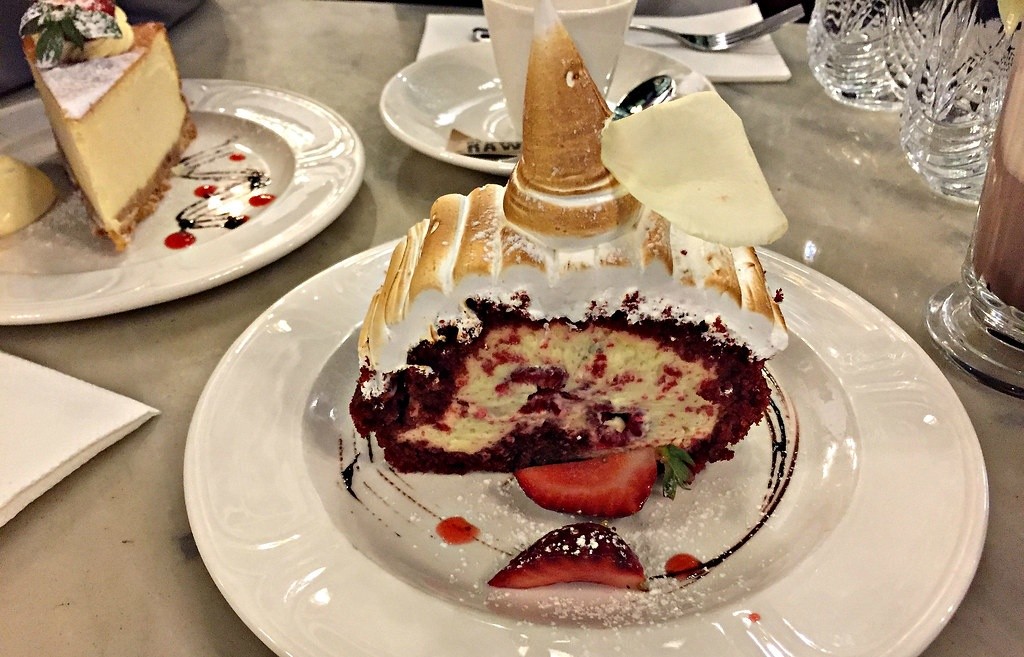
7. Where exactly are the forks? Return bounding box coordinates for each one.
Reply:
[471,4,804,51]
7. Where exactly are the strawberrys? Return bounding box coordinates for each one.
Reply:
[487,521,647,589]
[513,446,657,520]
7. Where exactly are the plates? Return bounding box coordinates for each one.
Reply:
[0,79,366,326]
[379,39,714,176]
[182,238,991,657]
[417,4,791,86]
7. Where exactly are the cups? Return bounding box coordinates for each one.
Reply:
[482,0,638,142]
[807,0,1024,401]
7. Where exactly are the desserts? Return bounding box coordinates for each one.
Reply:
[345,0,791,476]
[0,0,199,252]
